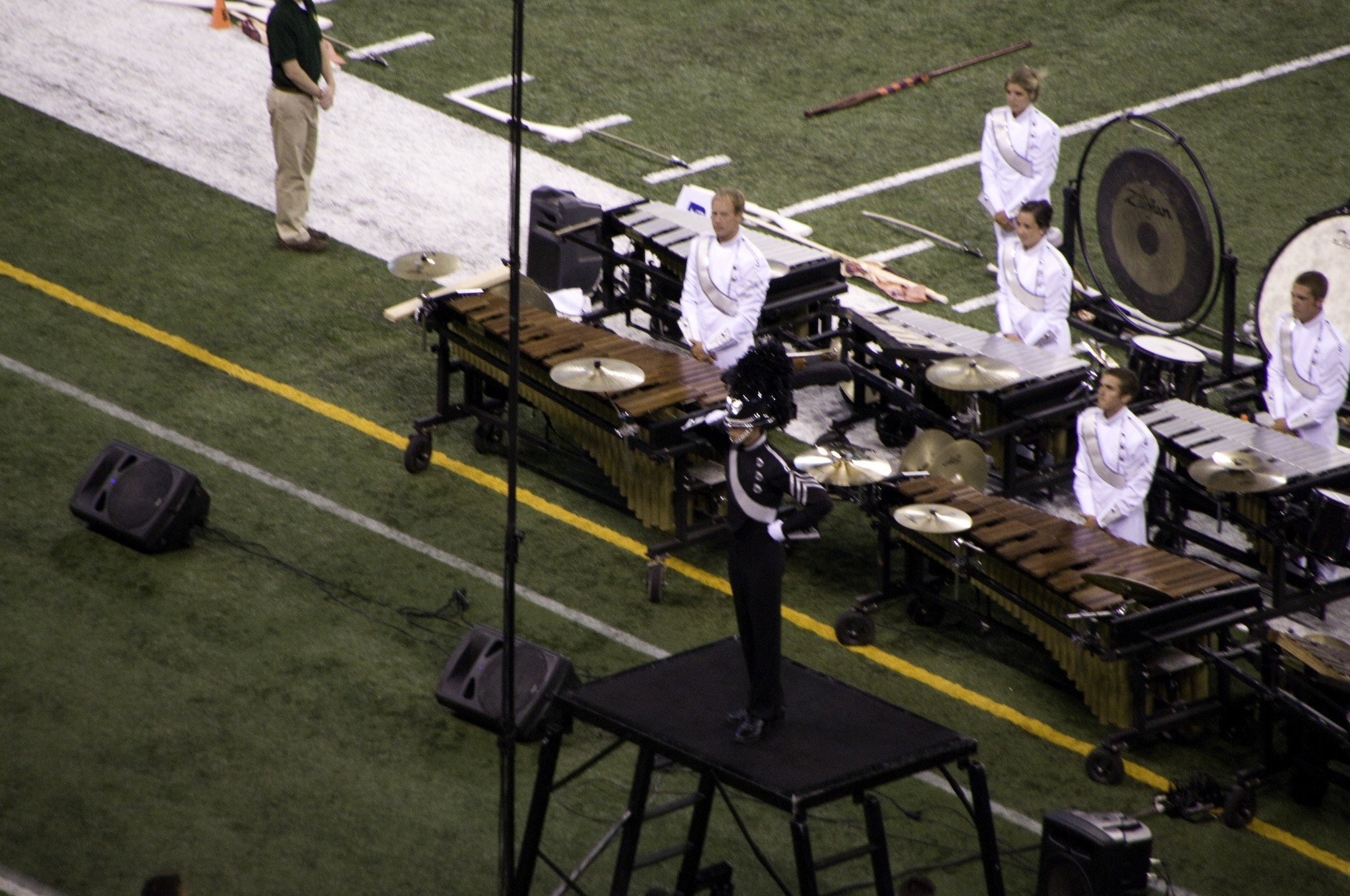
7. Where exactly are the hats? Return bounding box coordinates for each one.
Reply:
[723,392,774,425]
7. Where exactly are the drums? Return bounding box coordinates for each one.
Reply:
[1127,333,1207,401]
[1253,203,1350,362]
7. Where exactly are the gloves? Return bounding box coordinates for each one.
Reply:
[767,519,786,541]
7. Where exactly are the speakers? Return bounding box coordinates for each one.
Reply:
[433,624,582,743]
[69,437,210,555]
[1035,810,1153,896]
[525,185,604,292]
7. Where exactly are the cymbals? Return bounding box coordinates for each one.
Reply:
[926,357,1021,392]
[898,429,988,492]
[1188,451,1287,493]
[893,504,973,533]
[489,272,558,316]
[793,440,892,486]
[1081,572,1176,605]
[388,251,461,280]
[550,357,646,392]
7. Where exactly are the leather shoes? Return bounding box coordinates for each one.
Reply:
[726,708,749,724]
[736,713,772,745]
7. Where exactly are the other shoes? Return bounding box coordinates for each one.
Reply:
[308,230,324,239]
[275,234,327,253]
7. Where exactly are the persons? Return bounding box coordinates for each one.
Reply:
[992,200,1072,355]
[979,65,1061,244]
[1267,271,1349,585]
[678,189,768,376]
[266,0,336,253]
[723,387,834,743]
[1072,367,1162,546]
